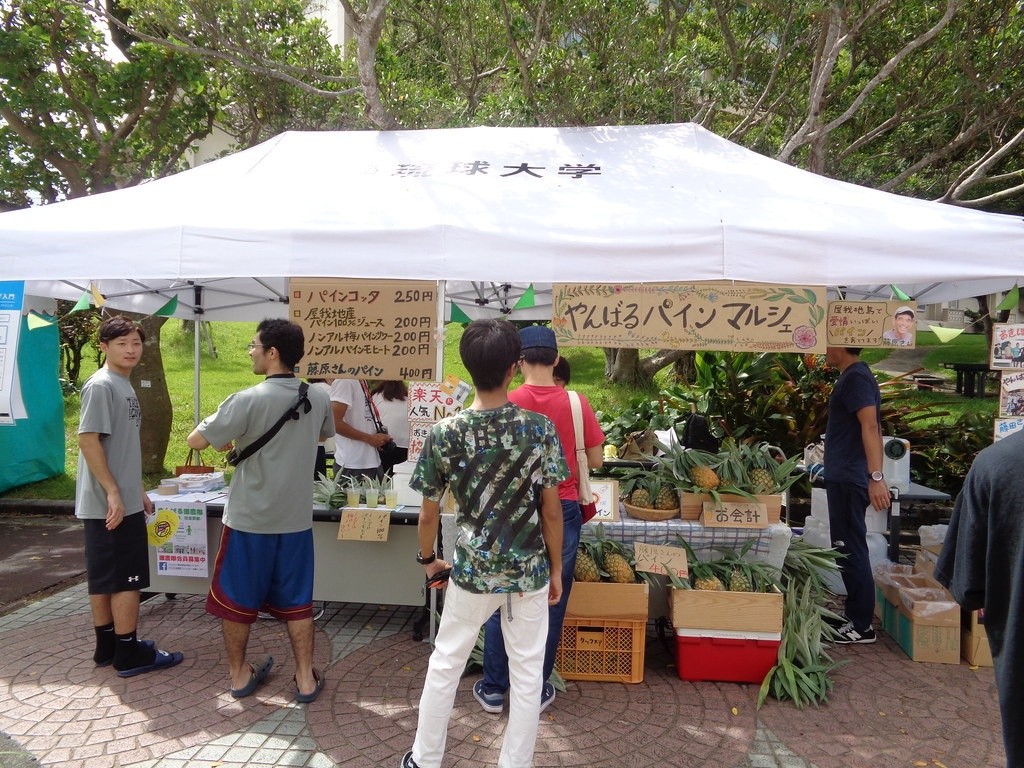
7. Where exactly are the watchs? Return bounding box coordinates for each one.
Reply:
[868,470,884,482]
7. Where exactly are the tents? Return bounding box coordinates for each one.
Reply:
[0,120,1024,475]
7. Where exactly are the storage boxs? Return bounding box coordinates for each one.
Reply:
[668,581,784,633]
[673,627,782,684]
[876,563,994,667]
[565,578,649,621]
[392,461,423,507]
[679,491,782,523]
[553,620,645,684]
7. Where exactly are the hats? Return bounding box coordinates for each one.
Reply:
[519,326,558,352]
[895,306,914,316]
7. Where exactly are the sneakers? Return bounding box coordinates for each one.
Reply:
[473,679,503,714]
[401,751,420,768]
[538,682,555,713]
[823,611,877,644]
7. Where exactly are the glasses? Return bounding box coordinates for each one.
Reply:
[248,344,265,350]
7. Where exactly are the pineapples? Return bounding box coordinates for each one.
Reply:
[569,428,811,590]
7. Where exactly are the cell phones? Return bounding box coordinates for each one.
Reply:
[424,569,453,588]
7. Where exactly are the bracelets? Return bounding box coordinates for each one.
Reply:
[416,549,436,565]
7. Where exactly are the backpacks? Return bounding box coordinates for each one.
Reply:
[680,413,718,454]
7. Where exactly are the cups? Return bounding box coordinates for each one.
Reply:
[365,488,379,508]
[385,489,398,509]
[348,488,361,508]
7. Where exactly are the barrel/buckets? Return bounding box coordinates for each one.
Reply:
[800,510,890,602]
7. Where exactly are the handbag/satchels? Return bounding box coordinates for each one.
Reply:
[574,449,598,525]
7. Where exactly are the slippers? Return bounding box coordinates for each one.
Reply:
[230,655,274,698]
[117,649,184,677]
[96,640,154,666]
[295,666,325,703]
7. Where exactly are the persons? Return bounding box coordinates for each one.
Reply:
[811,347,895,648]
[471,323,607,717]
[369,380,408,471]
[930,425,1024,768]
[71,313,185,676]
[327,378,393,496]
[553,354,572,392]
[184,316,341,706]
[301,378,337,488]
[882,305,915,347]
[396,316,566,768]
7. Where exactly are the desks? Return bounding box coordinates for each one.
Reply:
[577,501,792,586]
[136,483,443,650]
[794,458,952,564]
